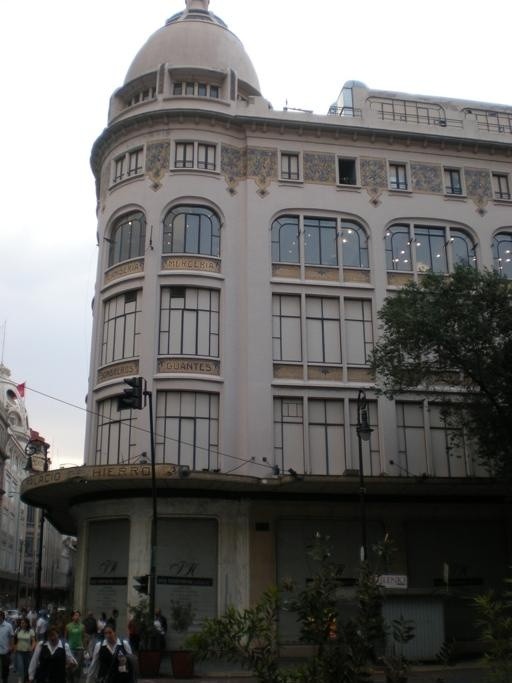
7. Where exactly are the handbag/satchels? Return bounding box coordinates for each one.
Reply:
[95,676,108,683]
[14,634,18,644]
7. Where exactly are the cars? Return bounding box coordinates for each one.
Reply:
[5,608,47,621]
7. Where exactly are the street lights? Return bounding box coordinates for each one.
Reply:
[23,436,50,606]
[350,385,378,679]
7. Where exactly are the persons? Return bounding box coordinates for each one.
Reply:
[0,599,167,683]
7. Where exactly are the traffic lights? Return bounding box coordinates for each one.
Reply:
[118,376,149,411]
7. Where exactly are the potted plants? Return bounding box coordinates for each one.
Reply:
[125,591,199,680]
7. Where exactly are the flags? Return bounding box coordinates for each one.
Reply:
[12,381,24,399]
